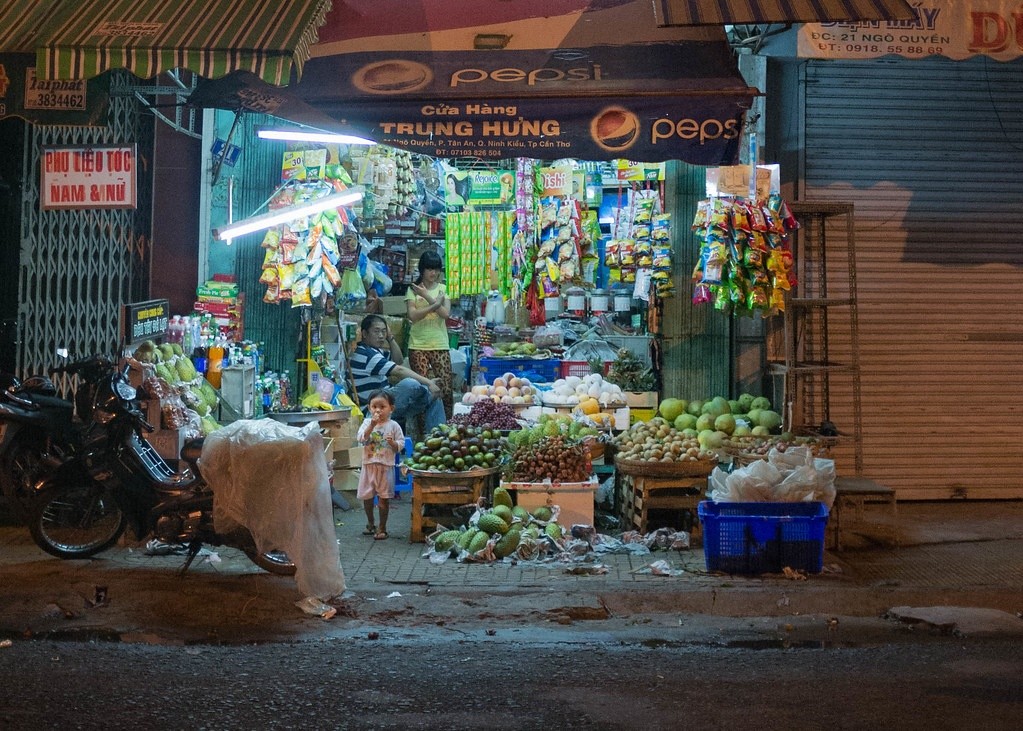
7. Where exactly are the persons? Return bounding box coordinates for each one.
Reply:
[446,172,472,206]
[404,251,453,421]
[356,390,405,542]
[344,315,447,432]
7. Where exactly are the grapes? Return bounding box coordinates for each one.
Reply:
[448,398,525,431]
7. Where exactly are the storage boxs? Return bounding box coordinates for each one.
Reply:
[220,365,256,422]
[322,422,351,438]
[542,407,556,414]
[698,500,828,574]
[333,470,361,490]
[515,405,542,421]
[400,220,415,235]
[322,437,334,463]
[717,164,771,202]
[630,409,658,425]
[499,472,601,536]
[405,275,412,282]
[345,314,367,342]
[328,358,344,373]
[372,237,386,247]
[479,358,560,386]
[377,296,408,315]
[321,325,344,343]
[370,247,410,284]
[333,437,351,451]
[601,406,631,431]
[379,315,405,350]
[705,162,781,198]
[333,446,363,469]
[459,345,471,380]
[625,391,659,410]
[413,275,419,282]
[557,408,570,414]
[323,343,343,360]
[453,401,474,415]
[193,273,245,342]
[342,321,359,342]
[385,220,401,235]
[561,360,613,379]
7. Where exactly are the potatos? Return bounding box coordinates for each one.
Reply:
[613,419,822,462]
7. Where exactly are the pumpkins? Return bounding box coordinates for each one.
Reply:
[572,398,616,428]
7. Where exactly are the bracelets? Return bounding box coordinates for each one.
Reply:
[387,336,396,342]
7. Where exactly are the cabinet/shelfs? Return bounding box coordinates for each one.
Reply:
[603,333,654,364]
[760,202,867,524]
[119,356,186,461]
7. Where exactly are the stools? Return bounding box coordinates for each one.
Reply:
[373,437,413,505]
[834,476,900,554]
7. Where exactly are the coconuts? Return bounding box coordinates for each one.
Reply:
[659,393,780,437]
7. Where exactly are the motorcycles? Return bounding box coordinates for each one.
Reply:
[0,352,120,527]
[31,359,352,577]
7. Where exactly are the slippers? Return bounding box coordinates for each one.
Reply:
[363,525,376,534]
[374,531,389,539]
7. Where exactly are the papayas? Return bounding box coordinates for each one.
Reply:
[495,343,537,356]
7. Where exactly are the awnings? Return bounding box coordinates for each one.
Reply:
[651,0,917,27]
[197,42,759,167]
[0,0,333,128]
[757,1,1023,62]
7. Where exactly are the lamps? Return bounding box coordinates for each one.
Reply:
[211,172,366,241]
[253,125,378,146]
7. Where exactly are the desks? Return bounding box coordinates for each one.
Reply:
[411,477,488,545]
[619,474,709,537]
[592,465,616,474]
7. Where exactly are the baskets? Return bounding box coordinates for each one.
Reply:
[737,451,769,465]
[560,360,618,382]
[591,442,605,461]
[479,355,560,386]
[613,453,719,476]
[721,435,773,455]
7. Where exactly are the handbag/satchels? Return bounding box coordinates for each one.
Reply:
[337,254,393,310]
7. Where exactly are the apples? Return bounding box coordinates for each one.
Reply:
[462,372,536,404]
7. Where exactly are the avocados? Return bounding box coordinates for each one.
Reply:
[404,424,507,471]
